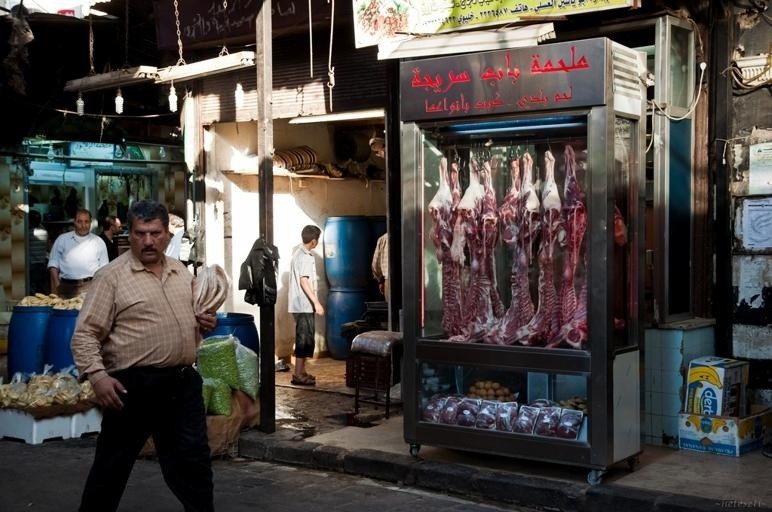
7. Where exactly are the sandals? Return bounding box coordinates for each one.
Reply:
[303,370,315,380]
[291,374,315,385]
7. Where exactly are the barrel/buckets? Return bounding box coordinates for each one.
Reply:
[323,215,375,292]
[7,306,53,383]
[200,312,260,357]
[326,291,370,361]
[47,308,79,374]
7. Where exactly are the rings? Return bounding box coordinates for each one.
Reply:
[207,314,212,321]
[203,327,208,332]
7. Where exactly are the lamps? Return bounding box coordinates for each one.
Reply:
[114,89,124,114]
[154,0,255,84]
[159,146,166,158]
[115,145,122,158]
[76,93,84,115]
[167,80,178,112]
[64,15,158,93]
[47,141,55,159]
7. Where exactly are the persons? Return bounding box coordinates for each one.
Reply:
[372,232,388,304]
[288,225,325,386]
[70,199,217,512]
[99,215,122,263]
[47,209,110,298]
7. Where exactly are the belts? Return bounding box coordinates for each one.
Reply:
[60,276,93,283]
